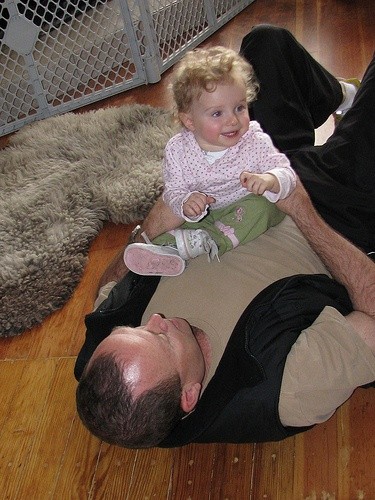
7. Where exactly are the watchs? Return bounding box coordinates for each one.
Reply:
[129,225,141,244]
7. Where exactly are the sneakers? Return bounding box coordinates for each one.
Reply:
[123,242,186,277]
[175,229,223,264]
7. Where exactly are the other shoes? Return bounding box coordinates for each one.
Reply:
[333,76,361,129]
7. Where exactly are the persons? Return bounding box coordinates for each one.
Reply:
[74,24,375,449]
[124,46,297,277]
